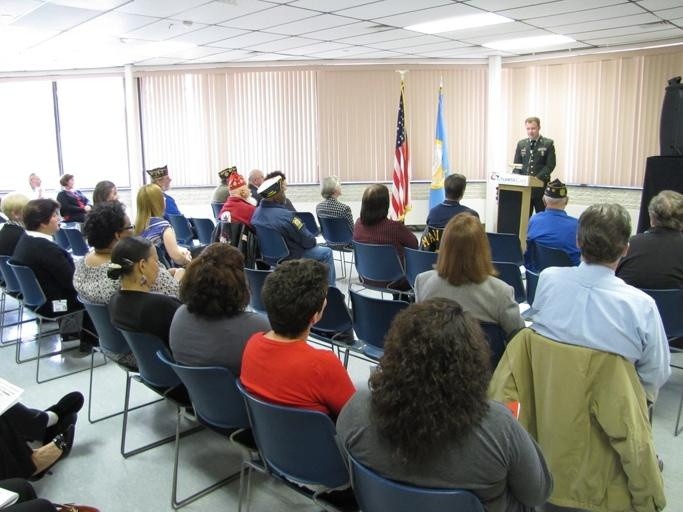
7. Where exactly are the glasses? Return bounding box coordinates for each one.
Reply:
[120,224,135,230]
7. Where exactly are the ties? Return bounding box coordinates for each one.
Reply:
[531,139,535,149]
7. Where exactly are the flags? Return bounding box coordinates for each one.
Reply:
[429,88,450,210]
[391,81,412,222]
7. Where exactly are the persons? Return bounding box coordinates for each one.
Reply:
[615,190,683,350]
[335,297,554,512]
[527,179,581,266]
[353,184,418,303]
[427,174,479,229]
[511,117,556,218]
[1,166,355,511]
[532,203,672,473]
[414,212,526,342]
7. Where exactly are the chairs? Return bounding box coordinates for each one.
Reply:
[155,350,267,511]
[76,296,203,454]
[233,382,354,511]
[46,214,682,435]
[109,321,254,503]
[342,442,484,511]
[1,257,81,364]
[7,259,105,384]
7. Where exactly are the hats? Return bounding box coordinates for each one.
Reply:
[147,165,167,179]
[218,166,246,190]
[544,178,567,198]
[257,176,282,198]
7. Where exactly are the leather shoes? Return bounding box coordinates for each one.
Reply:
[29,392,84,480]
